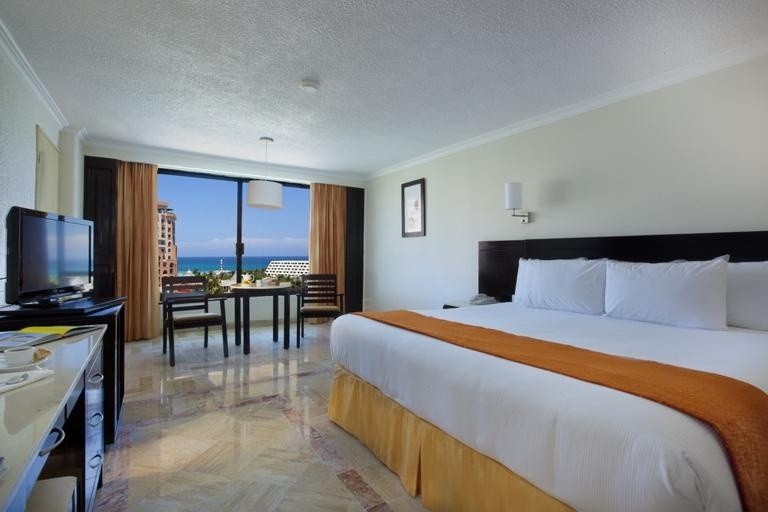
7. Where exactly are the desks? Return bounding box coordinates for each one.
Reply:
[230,280,295,355]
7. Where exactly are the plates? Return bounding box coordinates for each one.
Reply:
[0,347,52,370]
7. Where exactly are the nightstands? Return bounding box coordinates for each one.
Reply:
[442,301,501,308]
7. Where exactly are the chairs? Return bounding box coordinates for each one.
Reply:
[162,276,229,367]
[296,274,345,348]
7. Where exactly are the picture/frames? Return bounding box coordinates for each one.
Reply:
[400,178,425,237]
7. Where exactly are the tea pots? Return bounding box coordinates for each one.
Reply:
[240,272,254,285]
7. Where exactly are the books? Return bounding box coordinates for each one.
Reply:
[0,325,105,353]
[268,280,291,286]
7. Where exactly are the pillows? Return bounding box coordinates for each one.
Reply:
[601,254,730,331]
[728,261,767,333]
[512,258,607,315]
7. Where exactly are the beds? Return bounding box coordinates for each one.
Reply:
[326,230,768,512]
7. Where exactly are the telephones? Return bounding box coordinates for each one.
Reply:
[469,294,496,305]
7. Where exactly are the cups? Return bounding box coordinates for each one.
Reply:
[4,346,35,365]
[256,280,262,287]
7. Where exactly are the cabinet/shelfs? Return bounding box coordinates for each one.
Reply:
[0,302,126,444]
[0,323,108,512]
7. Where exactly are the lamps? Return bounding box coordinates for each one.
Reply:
[246,136,283,208]
[503,182,530,224]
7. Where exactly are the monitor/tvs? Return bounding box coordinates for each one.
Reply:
[5,204,96,310]
